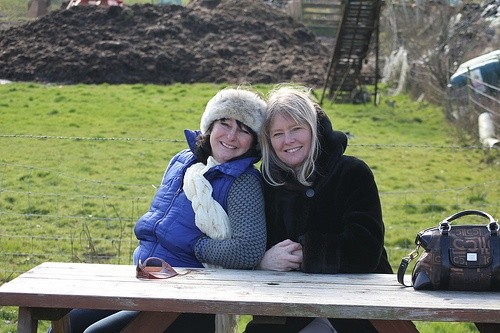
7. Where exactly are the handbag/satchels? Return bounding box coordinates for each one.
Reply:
[398,210,500,291]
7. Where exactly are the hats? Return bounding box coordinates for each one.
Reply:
[200,89,267,143]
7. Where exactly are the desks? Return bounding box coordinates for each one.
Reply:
[0,261,500,333]
